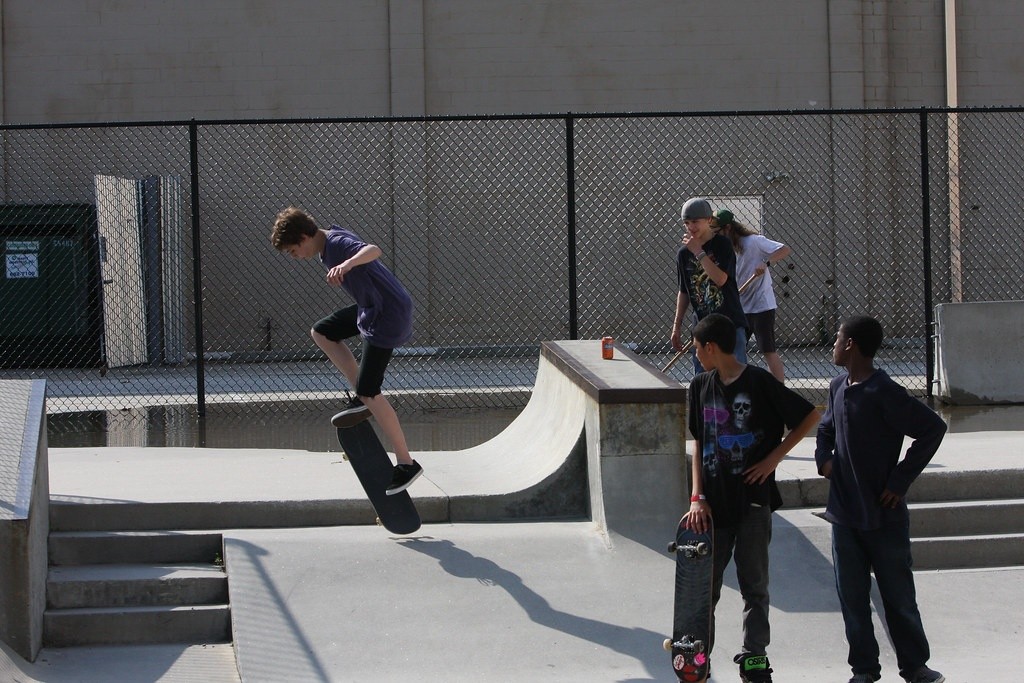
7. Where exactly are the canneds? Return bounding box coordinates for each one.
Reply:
[602,337,613,359]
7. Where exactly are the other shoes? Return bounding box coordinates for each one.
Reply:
[739,655,773,683]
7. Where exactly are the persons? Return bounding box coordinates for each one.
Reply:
[677,313,820,683]
[815,315,946,683]
[671,198,792,385]
[270,206,424,496]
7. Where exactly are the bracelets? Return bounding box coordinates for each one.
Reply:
[691,494,706,502]
[761,259,771,266]
[695,250,706,261]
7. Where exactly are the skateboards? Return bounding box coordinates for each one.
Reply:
[660,508,716,682]
[338,416,422,535]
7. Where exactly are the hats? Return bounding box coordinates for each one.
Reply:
[681,198,713,220]
[714,209,735,229]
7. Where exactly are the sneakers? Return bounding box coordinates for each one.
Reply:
[385,459,423,495]
[330,390,372,428]
[899,664,945,683]
[848,674,874,683]
